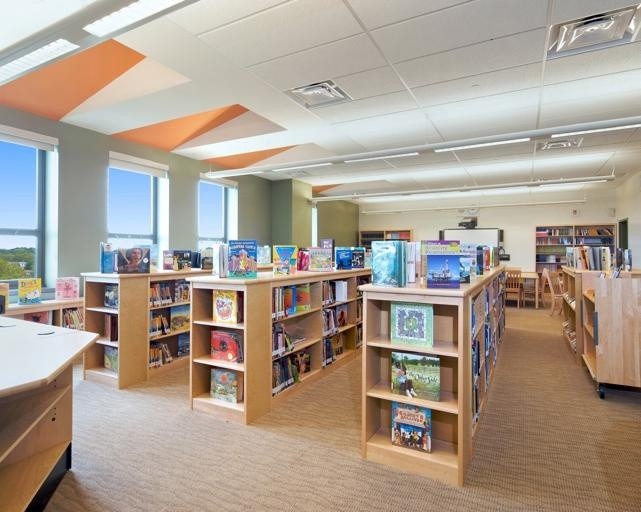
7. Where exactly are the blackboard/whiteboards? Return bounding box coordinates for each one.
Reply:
[438,228,504,248]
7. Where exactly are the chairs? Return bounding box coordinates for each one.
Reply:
[505,266,563,316]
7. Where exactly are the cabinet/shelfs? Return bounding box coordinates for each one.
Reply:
[357,265,506,489]
[80,268,213,388]
[186,268,371,426]
[561,264,582,363]
[581,272,641,399]
[534,223,617,293]
[359,227,413,250]
[0,297,85,364]
[0,315,100,512]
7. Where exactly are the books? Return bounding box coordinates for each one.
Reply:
[24,307,84,331]
[271,275,368,397]
[390,301,441,452]
[471,271,504,426]
[18,278,41,305]
[55,277,79,301]
[361,232,410,268]
[104,284,118,372]
[100,240,213,273]
[210,289,243,404]
[150,279,190,368]
[536,227,632,274]
[213,239,367,280]
[562,291,598,353]
[371,241,494,289]
